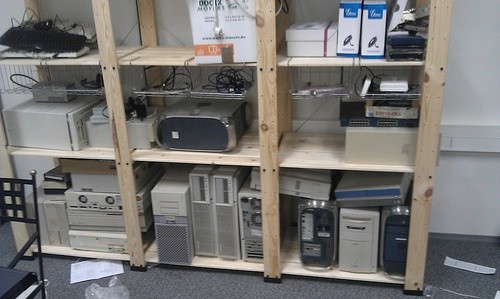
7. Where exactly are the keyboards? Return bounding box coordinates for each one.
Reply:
[0,45,90,59]
[0,26,87,54]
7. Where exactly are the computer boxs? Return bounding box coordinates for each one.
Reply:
[1,91,99,151]
[210,166,249,260]
[149,164,195,267]
[63,187,152,213]
[250,168,335,201]
[45,194,69,247]
[71,162,149,194]
[334,171,413,208]
[380,207,411,279]
[298,198,338,272]
[23,183,49,246]
[338,206,381,275]
[186,164,219,257]
[152,99,251,153]
[63,206,152,233]
[238,175,286,263]
[65,230,130,254]
[85,103,160,150]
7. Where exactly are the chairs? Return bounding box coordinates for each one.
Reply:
[0,168,50,299]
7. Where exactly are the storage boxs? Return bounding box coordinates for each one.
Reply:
[344,127,418,167]
[337,0,363,58]
[188,0,257,64]
[360,0,387,58]
[286,20,338,58]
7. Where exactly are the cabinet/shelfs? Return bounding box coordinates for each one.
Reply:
[0,0,452,293]
